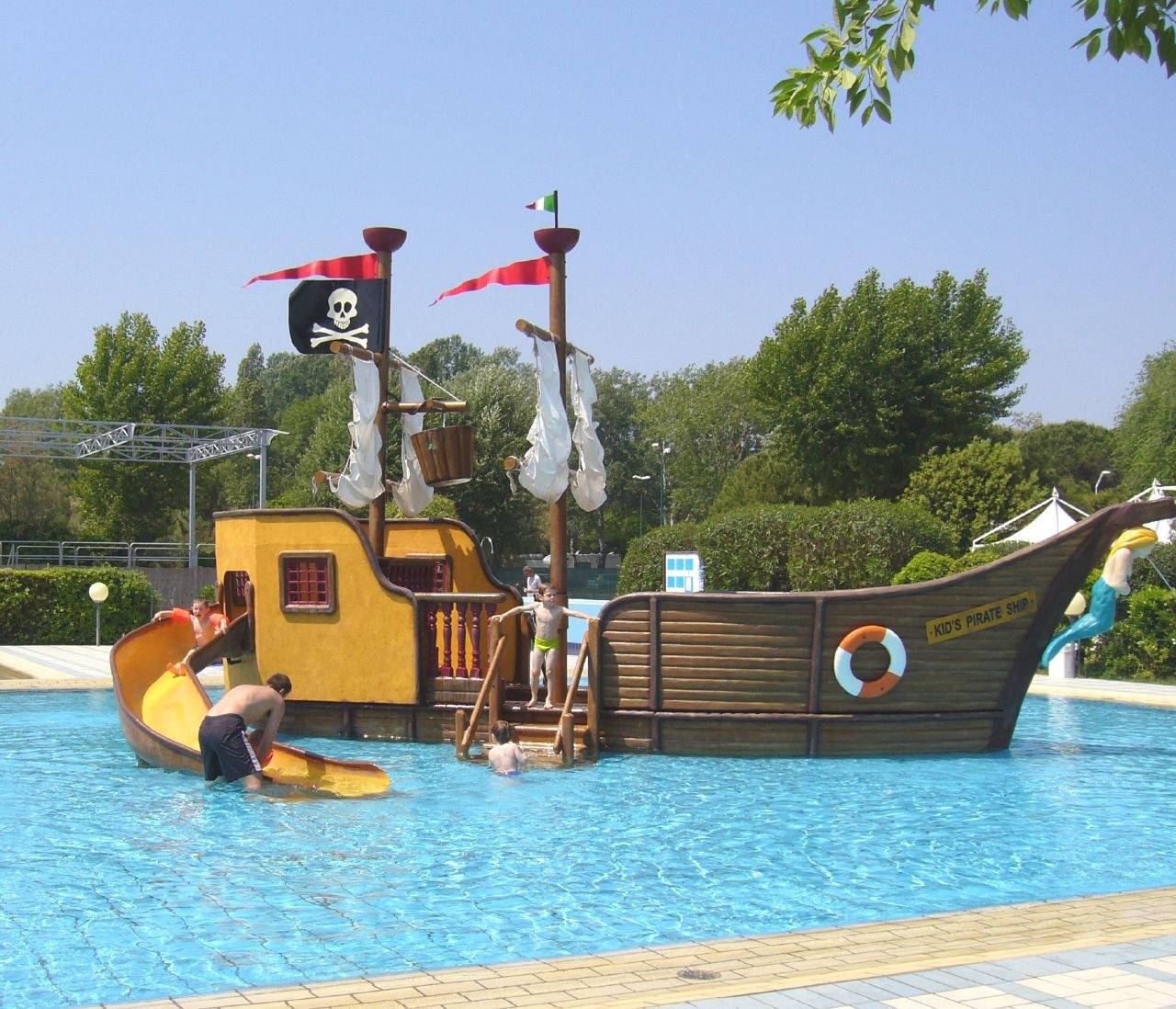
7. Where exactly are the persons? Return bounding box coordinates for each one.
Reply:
[1038,523,1158,671]
[488,721,528,777]
[488,582,602,709]
[523,566,543,597]
[152,596,228,676]
[198,673,292,792]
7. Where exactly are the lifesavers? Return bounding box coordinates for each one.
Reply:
[833,625,907,699]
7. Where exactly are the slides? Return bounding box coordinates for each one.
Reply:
[108,603,391,799]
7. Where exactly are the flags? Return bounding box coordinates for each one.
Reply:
[287,278,388,356]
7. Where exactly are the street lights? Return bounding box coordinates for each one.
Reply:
[651,439,672,526]
[631,475,652,537]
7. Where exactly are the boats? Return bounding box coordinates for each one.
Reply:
[108,225,1176,805]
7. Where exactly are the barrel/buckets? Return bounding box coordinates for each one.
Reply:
[410,397,477,487]
